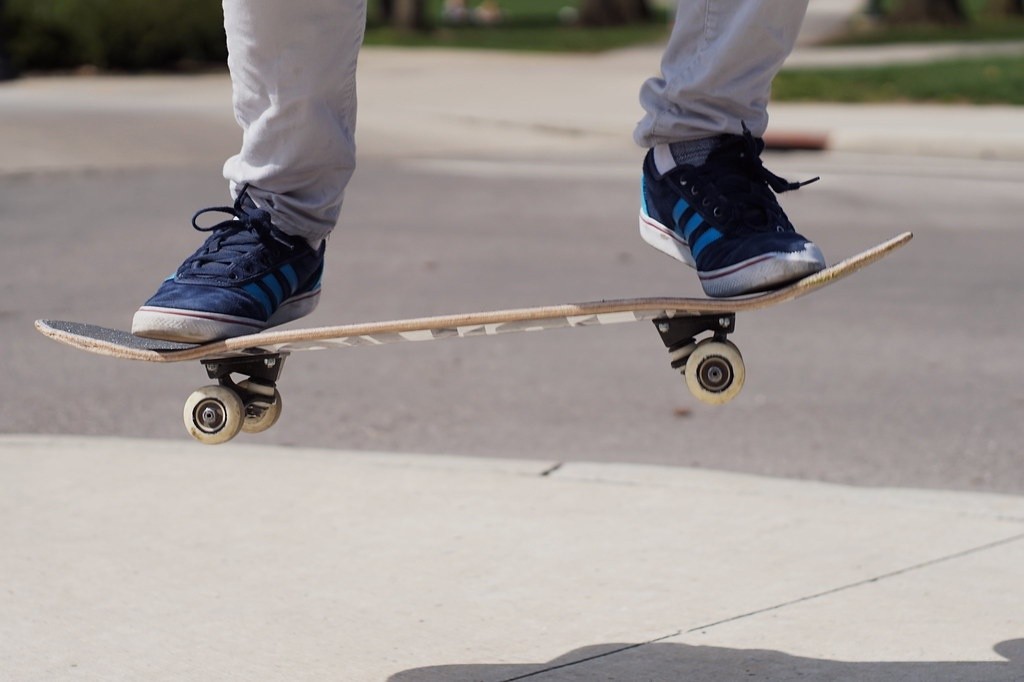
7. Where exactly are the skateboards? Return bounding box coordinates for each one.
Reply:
[33,231,917,446]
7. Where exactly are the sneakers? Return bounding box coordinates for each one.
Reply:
[131,183,326,342]
[639,133,827,299]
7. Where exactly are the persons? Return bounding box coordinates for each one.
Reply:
[132,1,827,344]
